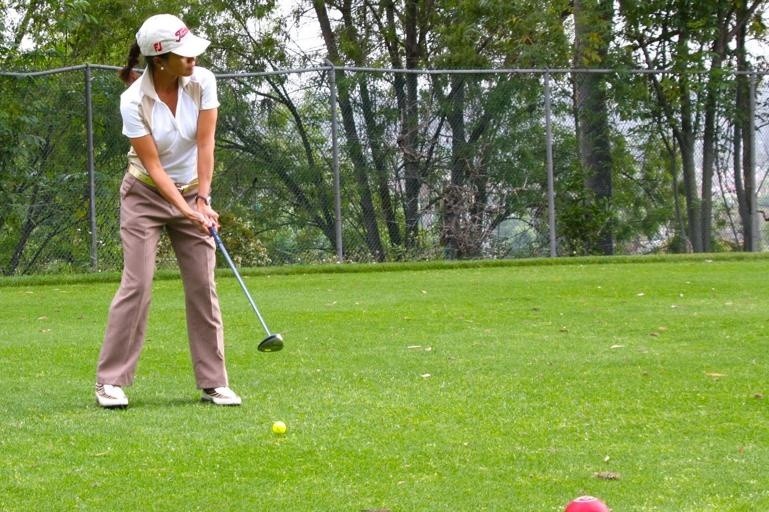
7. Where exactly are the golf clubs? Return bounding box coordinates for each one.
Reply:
[210,226,284,352]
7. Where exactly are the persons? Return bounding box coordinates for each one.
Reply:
[95,13,241,407]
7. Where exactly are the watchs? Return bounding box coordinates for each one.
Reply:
[196,194,212,206]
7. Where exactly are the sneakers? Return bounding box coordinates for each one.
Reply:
[200,385,242,406]
[94,381,130,408]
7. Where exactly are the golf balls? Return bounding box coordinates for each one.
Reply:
[272,421,286,433]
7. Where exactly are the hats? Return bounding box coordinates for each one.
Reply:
[134,13,212,57]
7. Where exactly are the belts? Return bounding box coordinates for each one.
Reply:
[128,163,200,194]
